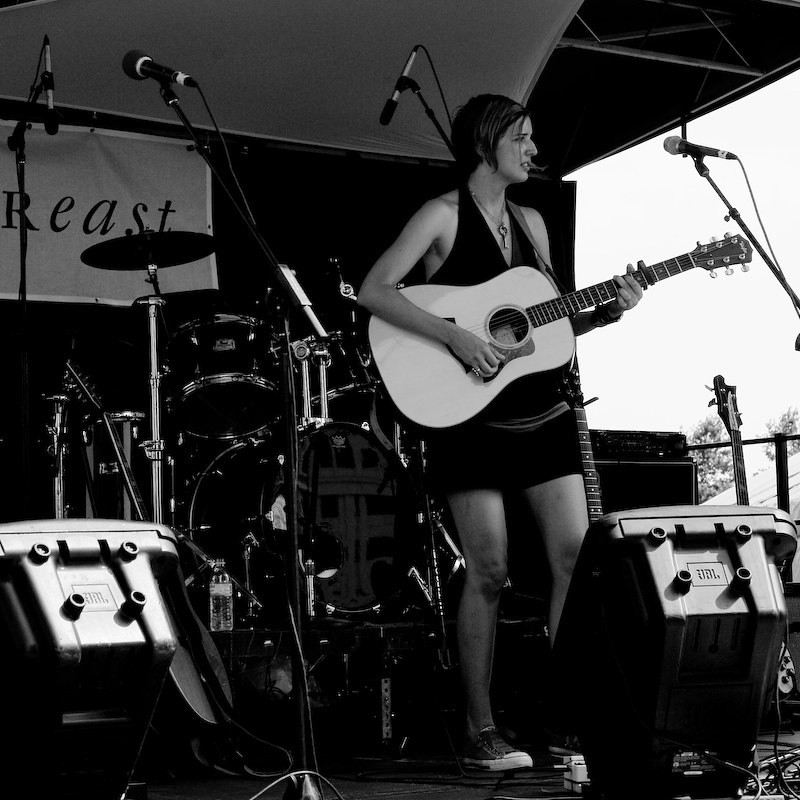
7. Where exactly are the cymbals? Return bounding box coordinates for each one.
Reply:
[80,230,216,272]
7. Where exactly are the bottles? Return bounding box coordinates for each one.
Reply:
[210,567,233,631]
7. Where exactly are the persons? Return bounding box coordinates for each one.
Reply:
[357,93,643,772]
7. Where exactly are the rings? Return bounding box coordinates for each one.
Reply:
[472,367,480,377]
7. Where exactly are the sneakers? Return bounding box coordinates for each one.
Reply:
[547,729,584,755]
[461,728,534,772]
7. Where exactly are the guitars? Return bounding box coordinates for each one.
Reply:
[560,365,606,526]
[702,372,751,506]
[63,358,150,522]
[365,230,756,435]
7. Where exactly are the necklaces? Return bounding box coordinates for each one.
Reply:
[467,184,510,250]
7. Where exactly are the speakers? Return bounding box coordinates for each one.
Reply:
[594,459,699,514]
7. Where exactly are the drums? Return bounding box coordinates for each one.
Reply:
[186,421,426,615]
[165,313,283,441]
[284,330,379,413]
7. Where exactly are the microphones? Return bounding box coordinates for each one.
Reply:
[663,136,738,161]
[380,44,420,126]
[43,34,59,136]
[121,50,198,88]
